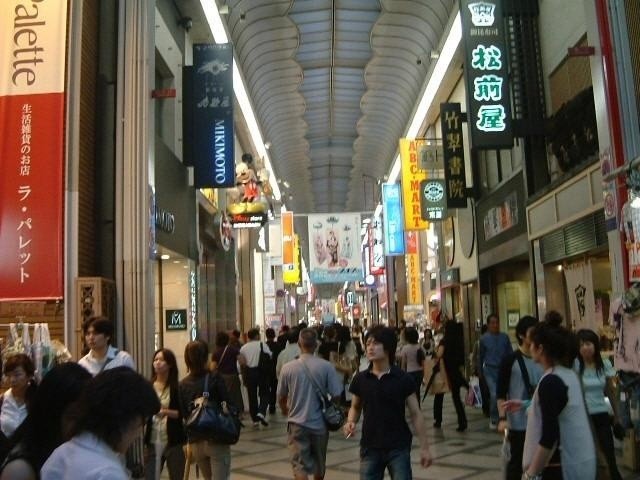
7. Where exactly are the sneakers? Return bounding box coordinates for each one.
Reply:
[433,421,442,429]
[456,421,467,432]
[251,412,269,427]
[488,418,497,431]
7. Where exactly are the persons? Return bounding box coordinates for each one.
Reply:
[236,154,263,185]
[0,310,625,480]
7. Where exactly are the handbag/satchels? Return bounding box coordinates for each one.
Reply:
[464,373,483,409]
[197,371,241,445]
[422,347,451,394]
[328,341,354,374]
[314,385,344,433]
[257,341,272,373]
[416,343,425,367]
